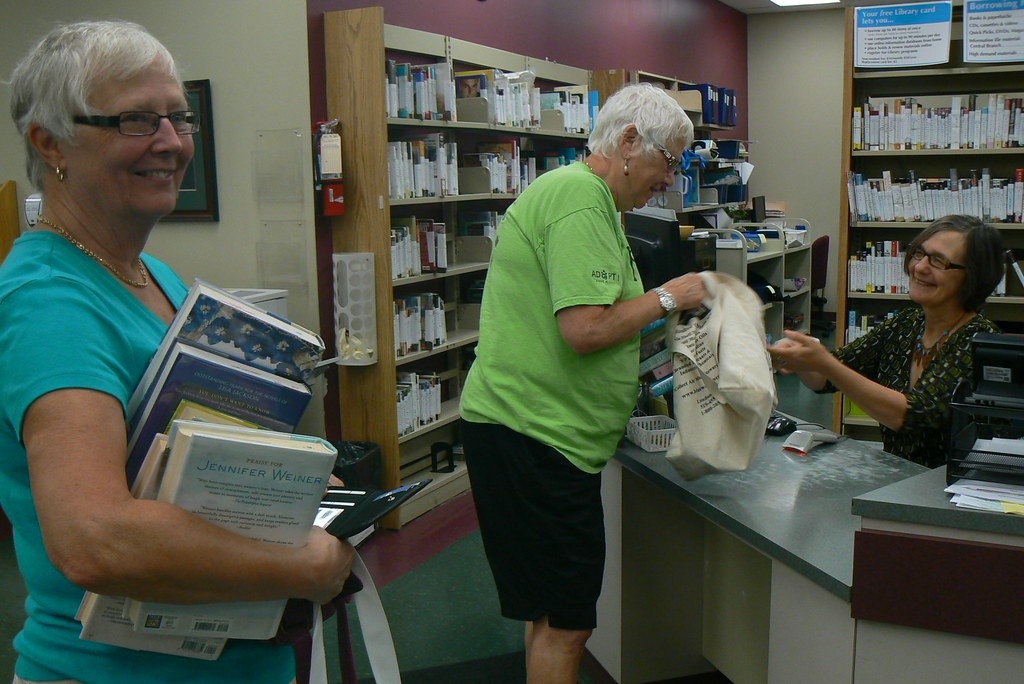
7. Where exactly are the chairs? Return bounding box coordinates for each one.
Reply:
[811,234,835,337]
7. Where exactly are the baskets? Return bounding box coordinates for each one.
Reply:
[627,415,678,453]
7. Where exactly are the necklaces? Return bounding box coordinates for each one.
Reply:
[583,161,593,171]
[913,311,967,367]
[37,215,148,288]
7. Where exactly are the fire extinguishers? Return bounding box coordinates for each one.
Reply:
[312,118,346,218]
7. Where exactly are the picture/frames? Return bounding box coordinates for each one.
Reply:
[159,78,218,223]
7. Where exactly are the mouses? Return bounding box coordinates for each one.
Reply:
[766,417,796,436]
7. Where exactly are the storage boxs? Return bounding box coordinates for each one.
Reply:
[783,229,806,242]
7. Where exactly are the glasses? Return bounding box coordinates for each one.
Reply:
[906,242,967,271]
[654,142,679,176]
[73,110,202,137]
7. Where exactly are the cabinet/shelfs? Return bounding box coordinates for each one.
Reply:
[323,6,1023,531]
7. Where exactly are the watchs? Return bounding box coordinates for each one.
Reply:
[651,287,677,320]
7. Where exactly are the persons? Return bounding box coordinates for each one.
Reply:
[767,214,1004,470]
[0,22,356,684]
[461,84,706,684]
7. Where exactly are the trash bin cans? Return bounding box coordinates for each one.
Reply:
[332,441,379,491]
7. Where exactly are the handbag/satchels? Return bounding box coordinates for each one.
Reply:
[662,268,774,483]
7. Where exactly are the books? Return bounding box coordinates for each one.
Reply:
[391,293,446,358]
[847,168,1024,223]
[395,371,441,437]
[847,240,1024,296]
[845,310,897,346]
[385,59,599,281]
[851,94,1024,150]
[74,278,378,661]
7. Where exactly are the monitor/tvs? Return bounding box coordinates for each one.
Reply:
[971,333,1024,410]
